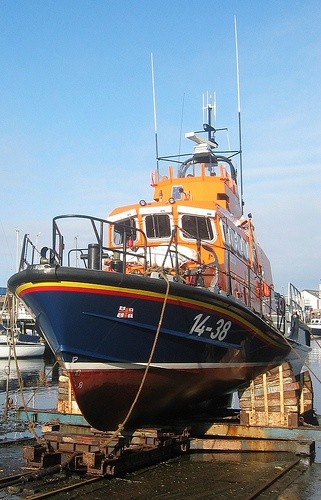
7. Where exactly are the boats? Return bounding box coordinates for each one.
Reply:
[0,334,44,359]
[305,319,321,337]
[7,14,313,439]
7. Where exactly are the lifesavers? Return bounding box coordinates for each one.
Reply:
[262,278,272,297]
[254,279,261,298]
[278,297,285,312]
